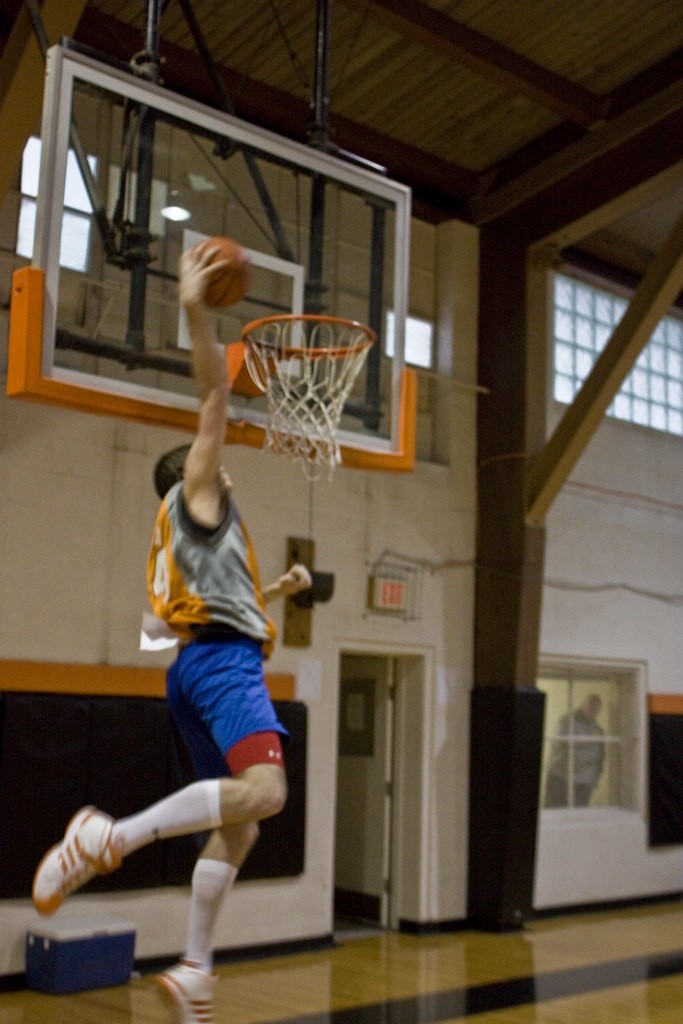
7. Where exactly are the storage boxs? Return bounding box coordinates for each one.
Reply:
[25,913,136,995]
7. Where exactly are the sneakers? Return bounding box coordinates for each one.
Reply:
[29,804,123,917]
[154,956,221,1024]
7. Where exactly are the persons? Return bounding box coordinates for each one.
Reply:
[544,694,605,809]
[33,242,311,1024]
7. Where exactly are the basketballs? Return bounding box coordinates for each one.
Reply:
[197,238,253,308]
